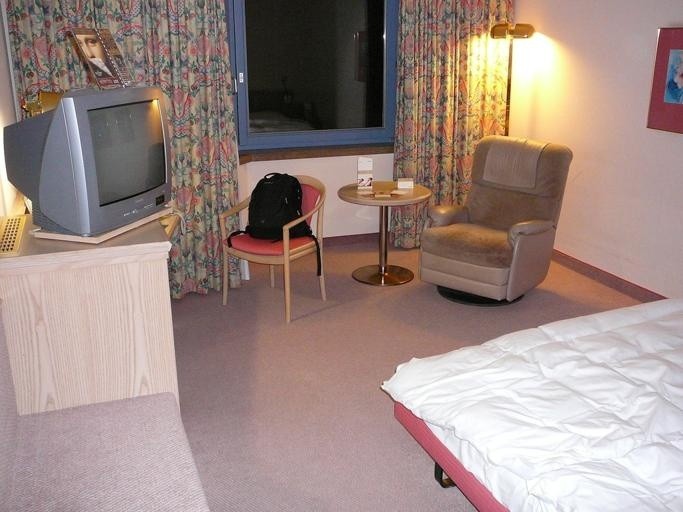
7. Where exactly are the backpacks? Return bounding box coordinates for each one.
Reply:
[245,172,312,240]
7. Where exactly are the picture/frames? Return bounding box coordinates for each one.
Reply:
[645,25,683,136]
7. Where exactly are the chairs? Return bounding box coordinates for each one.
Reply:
[418,135,573,308]
[218,174,327,323]
[0,314,208,511]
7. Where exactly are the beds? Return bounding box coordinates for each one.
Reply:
[381,298,683,512]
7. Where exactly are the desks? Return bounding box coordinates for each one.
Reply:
[337,181,432,286]
[0,192,180,416]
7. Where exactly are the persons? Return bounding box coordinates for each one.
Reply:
[75,33,115,79]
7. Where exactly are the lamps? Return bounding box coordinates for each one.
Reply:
[490,17,534,136]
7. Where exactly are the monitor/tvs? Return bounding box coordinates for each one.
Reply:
[4,88,172,243]
